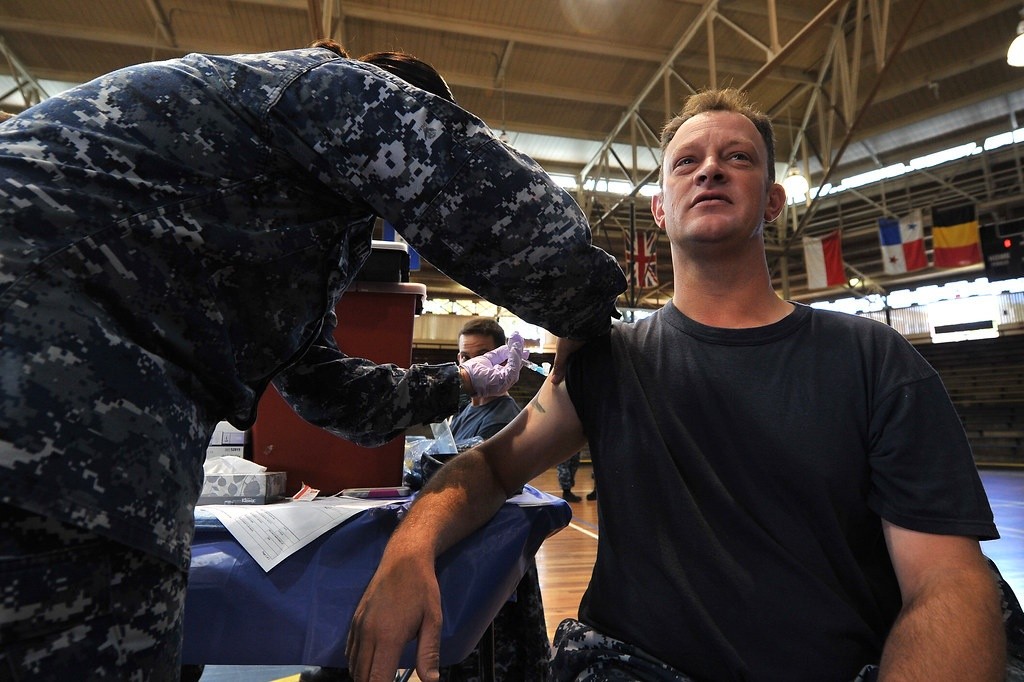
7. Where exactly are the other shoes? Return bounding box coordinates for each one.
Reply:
[562,492,581,502]
[587,491,596,501]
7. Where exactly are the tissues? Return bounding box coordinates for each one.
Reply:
[204,456,287,505]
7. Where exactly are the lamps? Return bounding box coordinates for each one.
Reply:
[1007,7,1024,67]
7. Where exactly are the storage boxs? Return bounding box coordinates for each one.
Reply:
[353,239,410,282]
[252,282,427,495]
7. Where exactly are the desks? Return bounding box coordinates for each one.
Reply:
[180,482,573,682]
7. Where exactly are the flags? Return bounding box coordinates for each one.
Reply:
[803,229,848,290]
[931,203,984,268]
[877,208,929,275]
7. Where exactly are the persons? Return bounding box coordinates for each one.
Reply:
[343,84,1011,682]
[586,471,597,501]
[0,39,629,682]
[556,451,582,502]
[405,315,522,491]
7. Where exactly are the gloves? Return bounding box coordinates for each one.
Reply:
[460,330,529,398]
